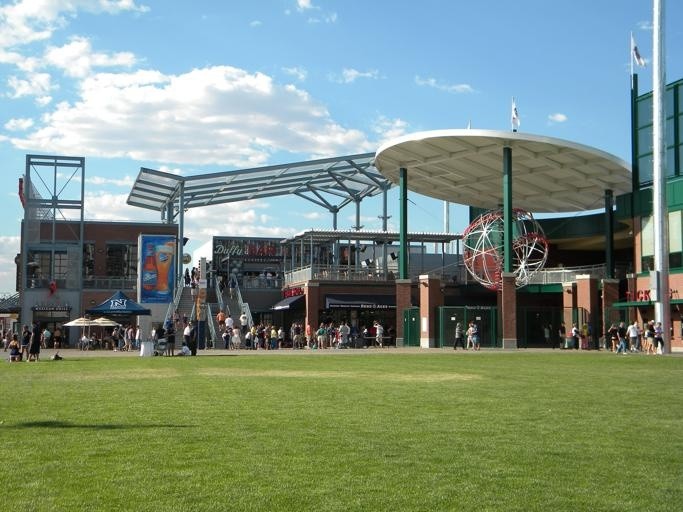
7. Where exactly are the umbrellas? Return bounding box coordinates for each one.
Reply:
[92,317,119,338]
[62,317,99,336]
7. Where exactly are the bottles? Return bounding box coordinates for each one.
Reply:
[142,242,158,292]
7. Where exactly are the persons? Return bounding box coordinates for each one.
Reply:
[557,321,566,349]
[215,310,224,332]
[572,323,582,350]
[469,321,481,351]
[465,322,475,351]
[173,309,179,332]
[238,312,249,333]
[607,319,665,355]
[452,322,468,350]
[541,319,552,344]
[1,321,393,364]
[184,267,235,299]
[223,315,233,330]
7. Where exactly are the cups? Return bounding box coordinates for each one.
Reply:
[152,245,174,295]
